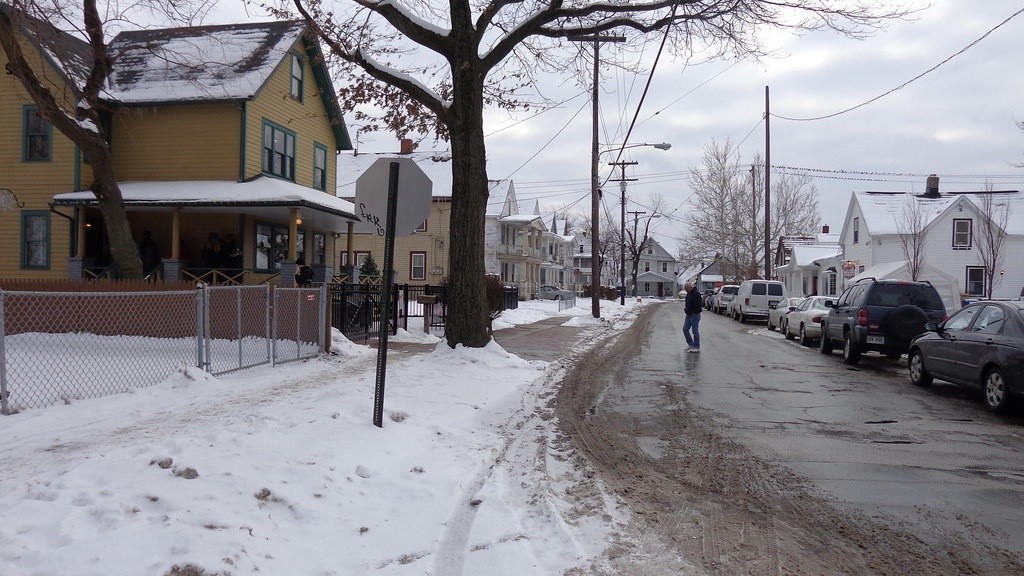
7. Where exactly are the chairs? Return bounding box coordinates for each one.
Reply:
[987,316,1002,331]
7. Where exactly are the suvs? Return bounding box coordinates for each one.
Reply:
[713,285,740,315]
[820,276,949,364]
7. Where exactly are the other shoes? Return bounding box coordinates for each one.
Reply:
[688,347,701,352]
[684,344,694,350]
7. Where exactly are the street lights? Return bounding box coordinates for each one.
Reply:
[592,143,672,318]
[621,215,661,306]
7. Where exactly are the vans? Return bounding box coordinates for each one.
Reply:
[732,279,787,323]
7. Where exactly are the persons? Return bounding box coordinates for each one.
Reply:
[682,282,703,353]
[202,231,242,270]
[140,230,158,272]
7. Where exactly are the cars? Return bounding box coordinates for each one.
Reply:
[767,296,848,346]
[907,300,1024,414]
[701,288,721,311]
[535,286,574,301]
[726,296,737,321]
